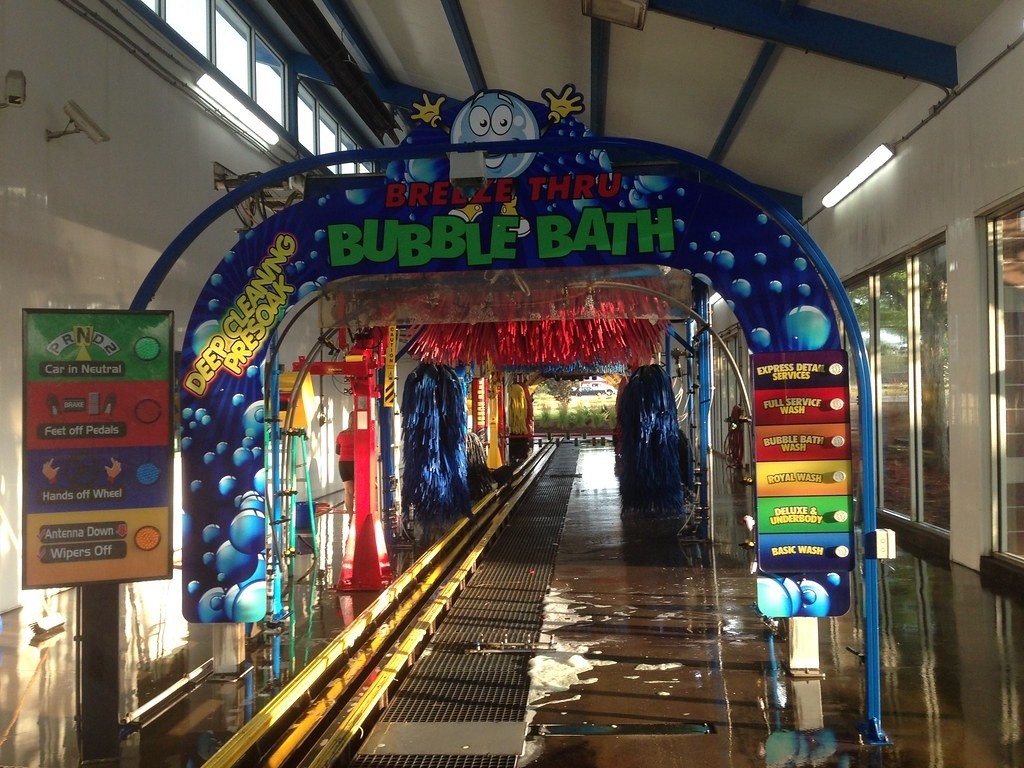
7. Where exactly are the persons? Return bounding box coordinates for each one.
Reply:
[335,411,355,529]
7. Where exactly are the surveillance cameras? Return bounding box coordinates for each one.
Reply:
[6,70,26,106]
[63,99,110,145]
